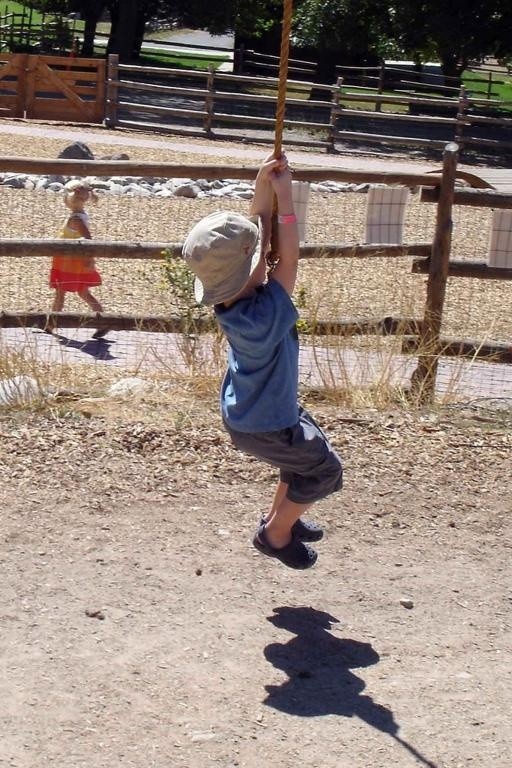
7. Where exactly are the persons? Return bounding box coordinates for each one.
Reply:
[179,146,344,572]
[42,179,112,339]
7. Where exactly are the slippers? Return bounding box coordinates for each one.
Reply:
[260,514,324,542]
[252,523,317,571]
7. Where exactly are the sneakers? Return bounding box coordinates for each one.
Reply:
[91,328,109,338]
[43,318,54,332]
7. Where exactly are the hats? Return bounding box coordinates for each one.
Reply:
[180,210,264,308]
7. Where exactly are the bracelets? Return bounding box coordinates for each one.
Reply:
[276,213,296,225]
